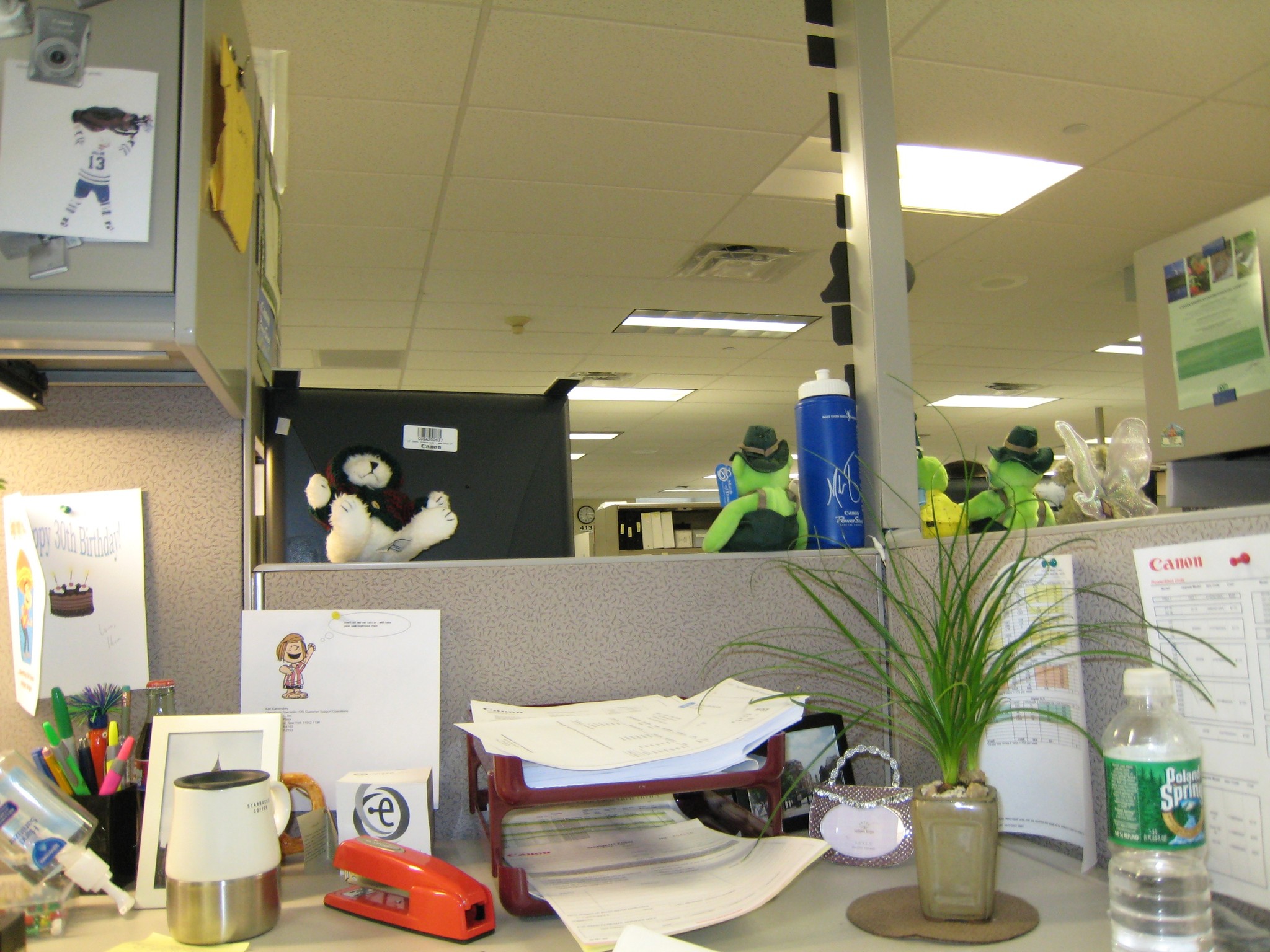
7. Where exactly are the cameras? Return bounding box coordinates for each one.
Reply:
[25,7,92,87]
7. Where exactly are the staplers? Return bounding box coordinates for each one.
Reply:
[324,837,494,945]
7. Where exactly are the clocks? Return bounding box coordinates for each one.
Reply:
[577,506,595,524]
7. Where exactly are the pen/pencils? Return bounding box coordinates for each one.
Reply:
[31,685,134,795]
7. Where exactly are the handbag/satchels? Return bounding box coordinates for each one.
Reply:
[810,746,916,868]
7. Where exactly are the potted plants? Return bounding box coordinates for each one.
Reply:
[698,372,1236,924]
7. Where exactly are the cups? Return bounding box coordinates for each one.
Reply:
[164,769,291,945]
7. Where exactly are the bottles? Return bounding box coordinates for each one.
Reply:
[795,369,865,549]
[1100,668,1215,952]
[133,678,178,883]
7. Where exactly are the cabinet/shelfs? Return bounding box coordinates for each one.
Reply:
[466,696,786,918]
[594,503,723,557]
[1,0,260,419]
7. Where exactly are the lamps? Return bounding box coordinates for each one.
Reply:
[1,361,48,412]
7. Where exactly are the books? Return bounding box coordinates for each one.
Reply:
[620,508,710,550]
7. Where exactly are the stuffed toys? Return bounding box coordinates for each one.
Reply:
[701,424,808,552]
[955,423,1058,533]
[908,414,968,538]
[305,445,458,563]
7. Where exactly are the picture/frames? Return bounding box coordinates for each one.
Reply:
[732,712,856,832]
[135,713,284,909]
